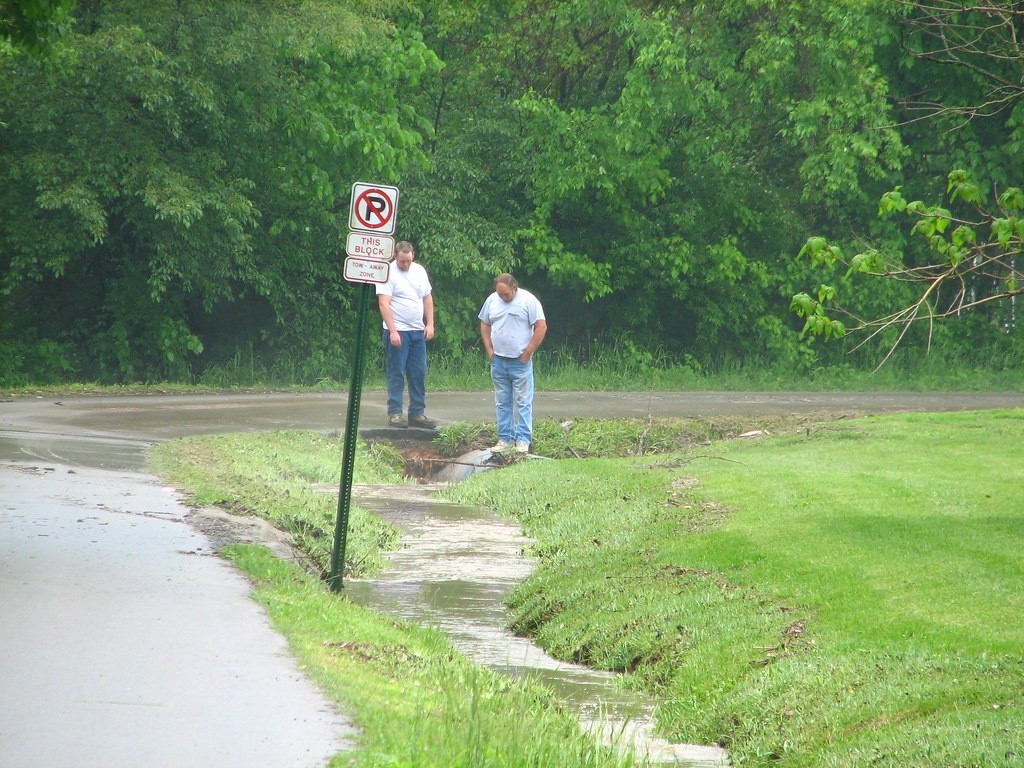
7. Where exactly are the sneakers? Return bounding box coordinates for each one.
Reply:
[388,414,408,428]
[490,439,510,453]
[515,440,529,452]
[408,415,437,428]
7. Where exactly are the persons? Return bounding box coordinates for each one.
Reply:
[374,240,439,429]
[478,273,547,453]
